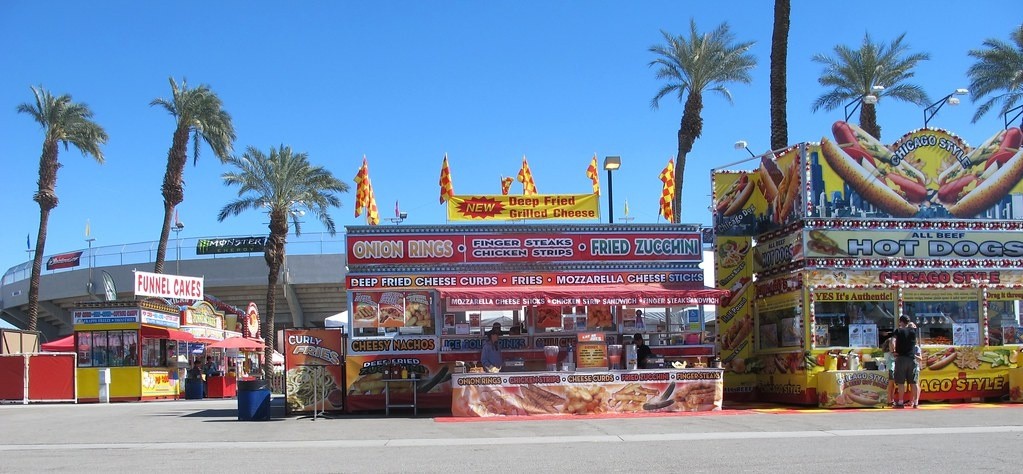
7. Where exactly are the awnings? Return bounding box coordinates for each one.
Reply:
[141,325,194,362]
[435,285,731,306]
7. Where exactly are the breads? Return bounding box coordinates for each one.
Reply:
[589,306,613,328]
[405,304,431,327]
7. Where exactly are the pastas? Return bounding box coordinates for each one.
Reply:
[288,366,334,406]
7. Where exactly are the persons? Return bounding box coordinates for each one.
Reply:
[490,323,502,332]
[887,315,921,407]
[630,333,651,369]
[203,356,216,371]
[194,360,201,375]
[481,330,503,371]
[516,322,527,333]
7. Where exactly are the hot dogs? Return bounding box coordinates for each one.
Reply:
[821,121,927,217]
[717,175,754,216]
[721,313,754,351]
[847,388,879,405]
[938,126,1023,218]
[927,348,957,370]
[721,277,752,308]
[358,308,374,318]
[773,154,800,225]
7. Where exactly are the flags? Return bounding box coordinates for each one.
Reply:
[500,176,513,195]
[439,156,454,204]
[353,155,379,225]
[586,157,599,194]
[517,157,537,194]
[657,158,675,223]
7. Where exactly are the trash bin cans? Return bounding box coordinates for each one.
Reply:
[237,379,272,420]
[185,377,203,399]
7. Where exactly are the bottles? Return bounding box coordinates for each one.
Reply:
[382,364,417,380]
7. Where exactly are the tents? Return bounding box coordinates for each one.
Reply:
[324,310,348,333]
[41,333,90,352]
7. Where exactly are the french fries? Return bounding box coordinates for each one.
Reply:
[609,383,659,411]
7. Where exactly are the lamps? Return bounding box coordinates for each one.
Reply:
[400,212,408,222]
[939,317,944,324]
[844,94,878,122]
[924,88,969,110]
[925,95,959,124]
[603,155,622,223]
[734,141,756,158]
[931,317,935,325]
[922,317,929,324]
[916,317,921,324]
[846,85,885,108]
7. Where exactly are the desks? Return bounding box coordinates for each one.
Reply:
[817,370,890,409]
[185,378,207,399]
[294,363,344,421]
[450,367,726,417]
[207,375,237,398]
[381,378,421,416]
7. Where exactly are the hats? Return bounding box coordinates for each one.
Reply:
[900,315,910,323]
[634,333,642,341]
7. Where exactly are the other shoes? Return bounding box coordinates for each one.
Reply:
[893,404,904,409]
[888,402,897,407]
[913,404,918,408]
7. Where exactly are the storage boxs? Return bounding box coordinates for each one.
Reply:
[685,334,699,345]
[760,324,778,349]
[686,322,702,330]
[781,318,801,346]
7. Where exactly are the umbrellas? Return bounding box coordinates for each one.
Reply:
[203,336,263,378]
